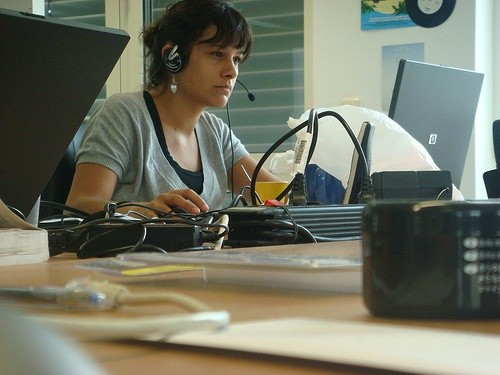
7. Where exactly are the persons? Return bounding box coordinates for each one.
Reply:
[62,0,288,226]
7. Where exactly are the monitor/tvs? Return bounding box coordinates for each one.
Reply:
[388,58,486,192]
[1,9,131,228]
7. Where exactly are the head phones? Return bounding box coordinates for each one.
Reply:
[151,1,185,73]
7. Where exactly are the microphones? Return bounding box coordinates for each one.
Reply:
[236,79,256,103]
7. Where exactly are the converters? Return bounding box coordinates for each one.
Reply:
[86,220,202,257]
